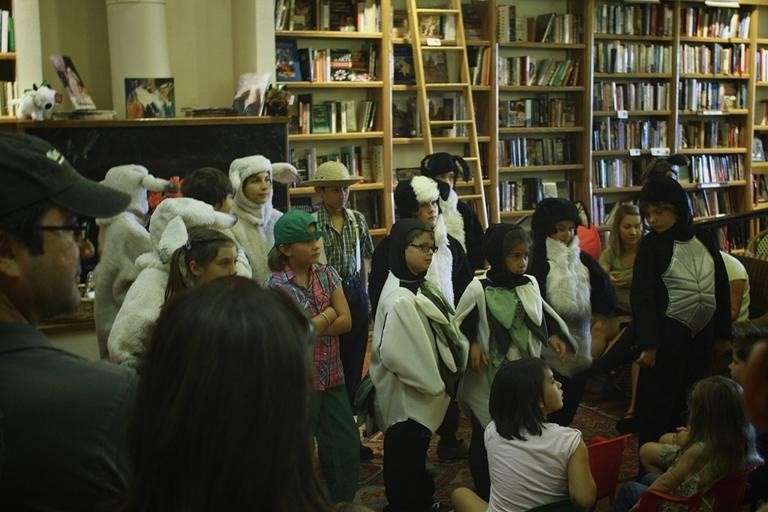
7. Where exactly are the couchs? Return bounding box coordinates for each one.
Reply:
[704,248,766,388]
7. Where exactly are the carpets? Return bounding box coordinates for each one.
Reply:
[308,392,640,512]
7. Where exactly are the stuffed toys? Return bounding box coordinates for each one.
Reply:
[17,83,57,122]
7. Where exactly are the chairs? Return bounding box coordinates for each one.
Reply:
[636,462,757,511]
[583,433,634,508]
[523,495,574,511]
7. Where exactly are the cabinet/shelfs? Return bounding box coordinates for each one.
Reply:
[591,0,677,251]
[676,0,754,253]
[273,1,390,249]
[498,0,592,229]
[0,0,20,119]
[391,0,497,231]
[754,1,768,241]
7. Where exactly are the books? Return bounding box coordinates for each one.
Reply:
[678,5,748,250]
[393,9,490,223]
[0,9,20,117]
[592,2,672,234]
[754,46,768,232]
[275,0,385,229]
[495,3,585,211]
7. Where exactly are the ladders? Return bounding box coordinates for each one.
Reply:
[405,0,489,234]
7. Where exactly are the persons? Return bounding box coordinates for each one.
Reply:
[268,162,375,512]
[126,77,174,118]
[362,149,483,512]
[450,197,614,512]
[74,81,94,106]
[596,175,768,512]
[129,274,334,512]
[93,151,299,372]
[1,130,137,512]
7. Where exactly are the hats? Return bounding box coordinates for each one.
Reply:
[229,155,298,225]
[420,152,470,182]
[532,197,578,221]
[301,160,364,187]
[94,164,177,225]
[134,197,238,270]
[1,129,132,219]
[484,222,531,284]
[267,209,325,257]
[395,175,450,212]
[637,152,694,233]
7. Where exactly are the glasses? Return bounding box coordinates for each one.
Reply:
[409,243,439,254]
[40,214,91,243]
